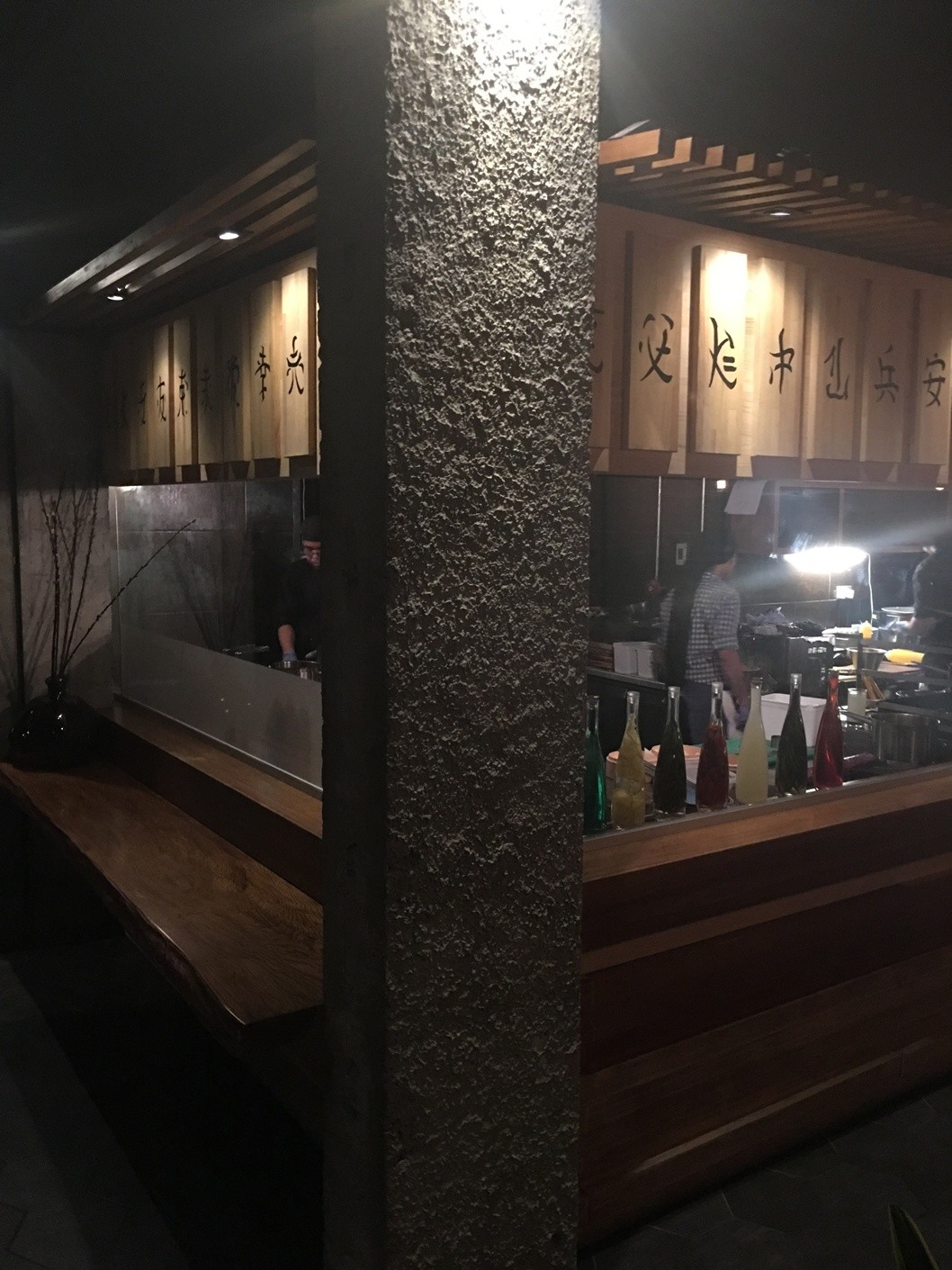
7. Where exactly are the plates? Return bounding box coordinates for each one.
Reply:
[881,606,916,614]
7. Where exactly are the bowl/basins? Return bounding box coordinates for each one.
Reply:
[846,646,887,670]
[624,601,663,619]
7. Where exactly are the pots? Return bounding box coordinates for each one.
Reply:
[267,660,322,682]
[838,708,940,769]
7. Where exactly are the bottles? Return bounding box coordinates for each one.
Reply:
[582,694,608,835]
[611,690,647,828]
[695,681,729,814]
[735,676,768,804]
[654,686,687,821]
[774,673,807,801]
[812,669,843,791]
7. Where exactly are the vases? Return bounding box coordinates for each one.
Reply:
[10,672,92,775]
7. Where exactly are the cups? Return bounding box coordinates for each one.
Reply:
[847,688,867,724]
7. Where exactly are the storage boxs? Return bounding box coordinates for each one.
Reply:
[761,693,827,749]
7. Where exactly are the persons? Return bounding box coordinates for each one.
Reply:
[896,543,952,682]
[276,515,317,662]
[659,526,752,746]
